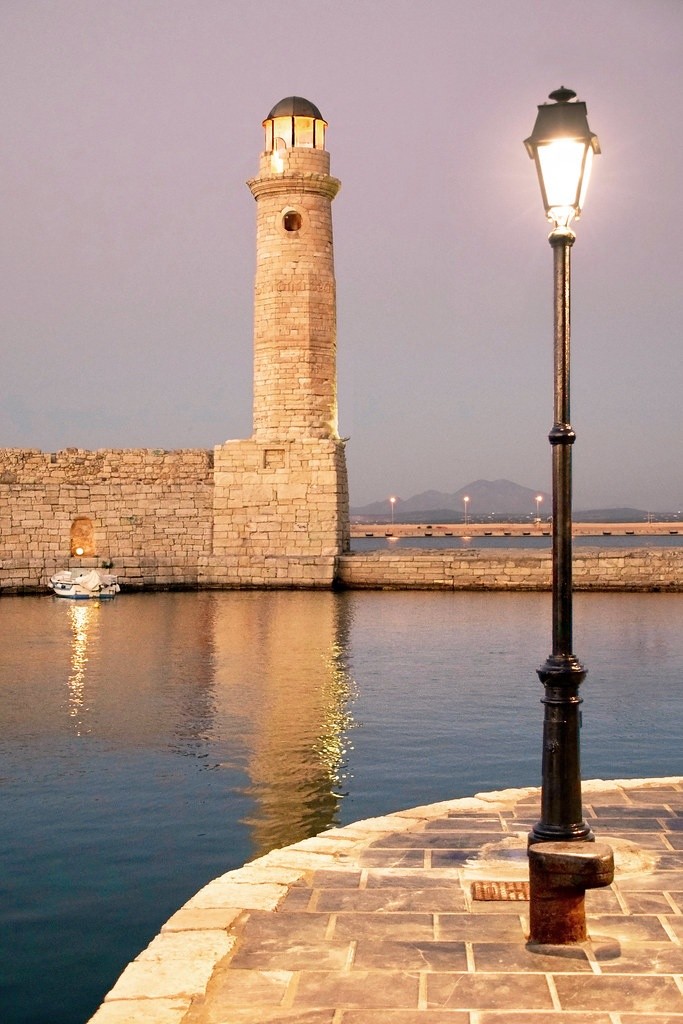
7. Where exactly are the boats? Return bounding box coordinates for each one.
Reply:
[48,571,121,599]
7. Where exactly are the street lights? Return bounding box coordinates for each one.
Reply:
[523,87,601,856]
[391,498,395,524]
[536,496,542,517]
[464,497,469,524]
